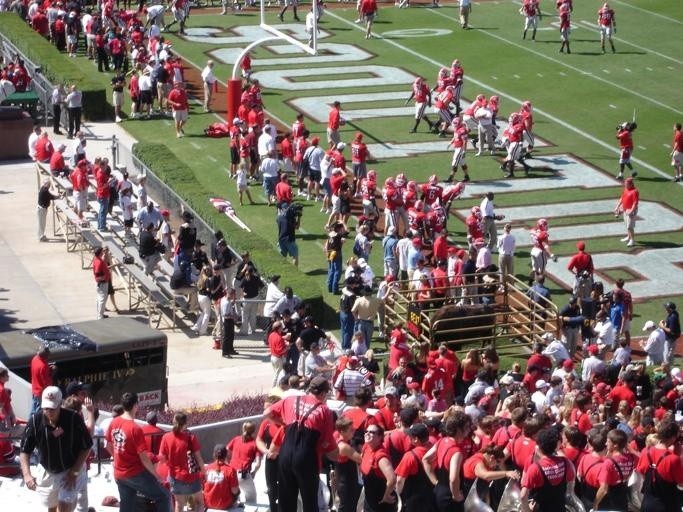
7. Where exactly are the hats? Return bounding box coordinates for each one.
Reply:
[40,239,681,440]
[226,116,362,201]
[31,127,170,219]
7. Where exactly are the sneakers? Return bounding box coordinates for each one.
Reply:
[614,170,682,183]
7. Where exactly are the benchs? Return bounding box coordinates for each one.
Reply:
[27,147,215,331]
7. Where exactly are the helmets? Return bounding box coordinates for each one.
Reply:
[367,59,549,233]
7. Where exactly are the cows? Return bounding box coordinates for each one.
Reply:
[430,302,497,355]
